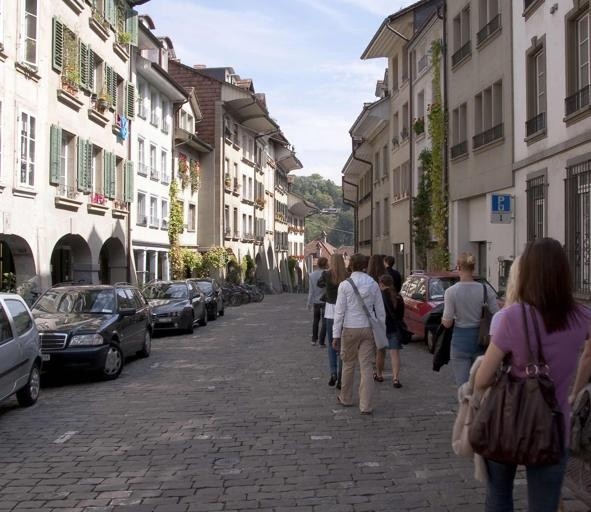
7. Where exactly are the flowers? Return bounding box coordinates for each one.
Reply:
[178,152,201,191]
[90,193,106,205]
[98,97,106,101]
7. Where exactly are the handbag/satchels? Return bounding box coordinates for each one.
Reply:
[478,315,491,344]
[399,323,409,344]
[469,372,565,467]
[372,320,389,349]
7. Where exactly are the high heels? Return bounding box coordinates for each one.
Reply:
[329,373,337,386]
[336,372,341,389]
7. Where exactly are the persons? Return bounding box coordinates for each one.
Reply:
[305,253,523,390]
[331,253,385,415]
[468,236,591,512]
[442,251,502,390]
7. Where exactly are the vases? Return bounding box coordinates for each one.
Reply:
[99,100,108,108]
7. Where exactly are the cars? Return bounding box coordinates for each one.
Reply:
[400,270,506,354]
[141,279,208,334]
[0,293,42,405]
[30,281,153,379]
[188,277,224,320]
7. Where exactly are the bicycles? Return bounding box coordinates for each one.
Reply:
[221,282,264,306]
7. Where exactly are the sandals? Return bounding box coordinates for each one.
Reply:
[373,375,383,382]
[393,379,402,388]
